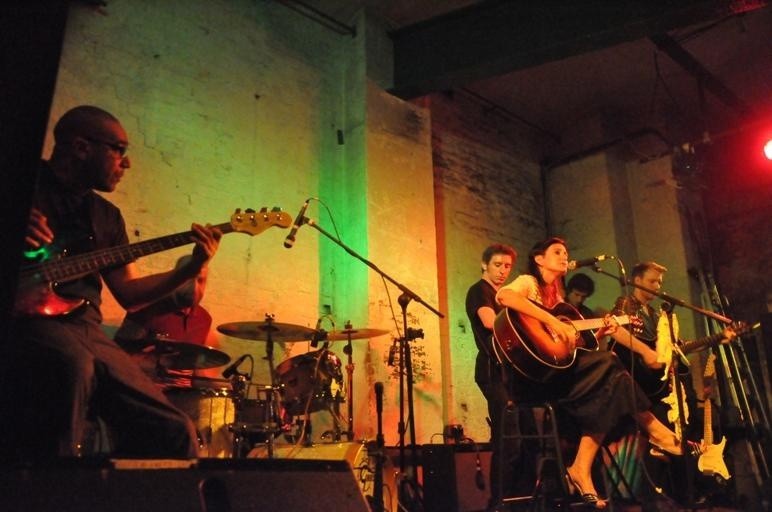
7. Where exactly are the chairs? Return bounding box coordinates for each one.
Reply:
[490,359,633,511]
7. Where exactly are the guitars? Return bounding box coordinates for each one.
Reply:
[493,303,643,380]
[697,389,731,480]
[0,208,291,319]
[607,321,753,397]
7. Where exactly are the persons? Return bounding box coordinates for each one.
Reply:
[540,504,541,507]
[494,236,683,512]
[607,260,672,446]
[555,273,607,506]
[113,253,222,436]
[460,245,543,502]
[5,105,199,467]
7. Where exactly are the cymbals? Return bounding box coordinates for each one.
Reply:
[217,322,318,341]
[125,340,230,369]
[325,328,390,340]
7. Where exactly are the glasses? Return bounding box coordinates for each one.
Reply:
[78,134,130,157]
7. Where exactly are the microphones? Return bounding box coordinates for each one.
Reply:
[222,353,248,379]
[283,201,309,250]
[568,253,606,270]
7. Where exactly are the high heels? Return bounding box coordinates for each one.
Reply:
[648,439,700,464]
[565,469,606,510]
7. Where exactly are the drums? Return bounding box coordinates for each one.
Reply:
[238,400,281,441]
[162,386,235,458]
[277,350,346,415]
